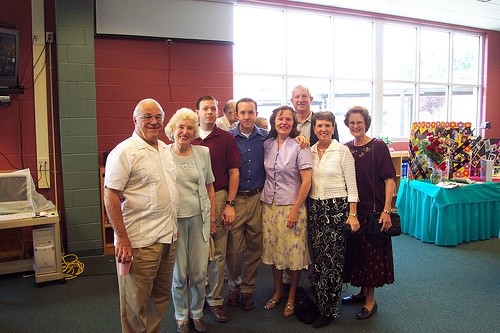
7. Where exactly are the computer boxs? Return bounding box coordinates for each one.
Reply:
[32,227,57,274]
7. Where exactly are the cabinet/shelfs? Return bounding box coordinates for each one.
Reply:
[99,165,116,256]
[390,151,409,213]
[0,212,63,284]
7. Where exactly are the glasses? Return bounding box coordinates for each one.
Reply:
[347,120,365,127]
[136,113,164,124]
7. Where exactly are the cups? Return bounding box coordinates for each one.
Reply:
[119,254,133,275]
[223,215,233,230]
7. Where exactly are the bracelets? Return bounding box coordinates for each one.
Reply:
[225,199,235,206]
[348,212,358,217]
[383,209,393,215]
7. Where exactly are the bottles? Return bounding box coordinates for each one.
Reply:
[480,159,494,182]
[401,157,409,178]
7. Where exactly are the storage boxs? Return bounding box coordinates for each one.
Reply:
[481,159,493,182]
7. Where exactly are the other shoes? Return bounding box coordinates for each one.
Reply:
[189,316,208,333]
[283,302,294,317]
[356,302,377,320]
[225,286,240,306]
[206,302,228,322]
[313,315,331,328]
[241,292,256,310]
[342,294,365,305]
[263,297,285,310]
[176,319,189,333]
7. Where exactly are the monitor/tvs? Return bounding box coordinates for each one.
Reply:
[0,172,34,214]
[0,28,19,88]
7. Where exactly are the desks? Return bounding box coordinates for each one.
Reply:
[395,175,500,245]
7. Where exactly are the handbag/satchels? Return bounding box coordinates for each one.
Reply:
[367,210,401,236]
[293,286,318,324]
[480,159,492,182]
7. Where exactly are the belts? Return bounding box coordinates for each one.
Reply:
[238,189,262,198]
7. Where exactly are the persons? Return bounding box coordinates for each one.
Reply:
[192,84,341,333]
[259,105,314,317]
[164,107,216,333]
[103,97,179,333]
[343,105,396,321]
[305,110,361,327]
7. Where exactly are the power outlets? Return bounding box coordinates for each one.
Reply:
[38,160,43,168]
[45,32,53,43]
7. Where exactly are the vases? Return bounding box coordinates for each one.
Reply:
[431,168,444,184]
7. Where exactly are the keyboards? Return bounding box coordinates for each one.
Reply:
[0,212,36,221]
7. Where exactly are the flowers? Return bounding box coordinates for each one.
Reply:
[378,136,395,153]
[410,131,449,172]
